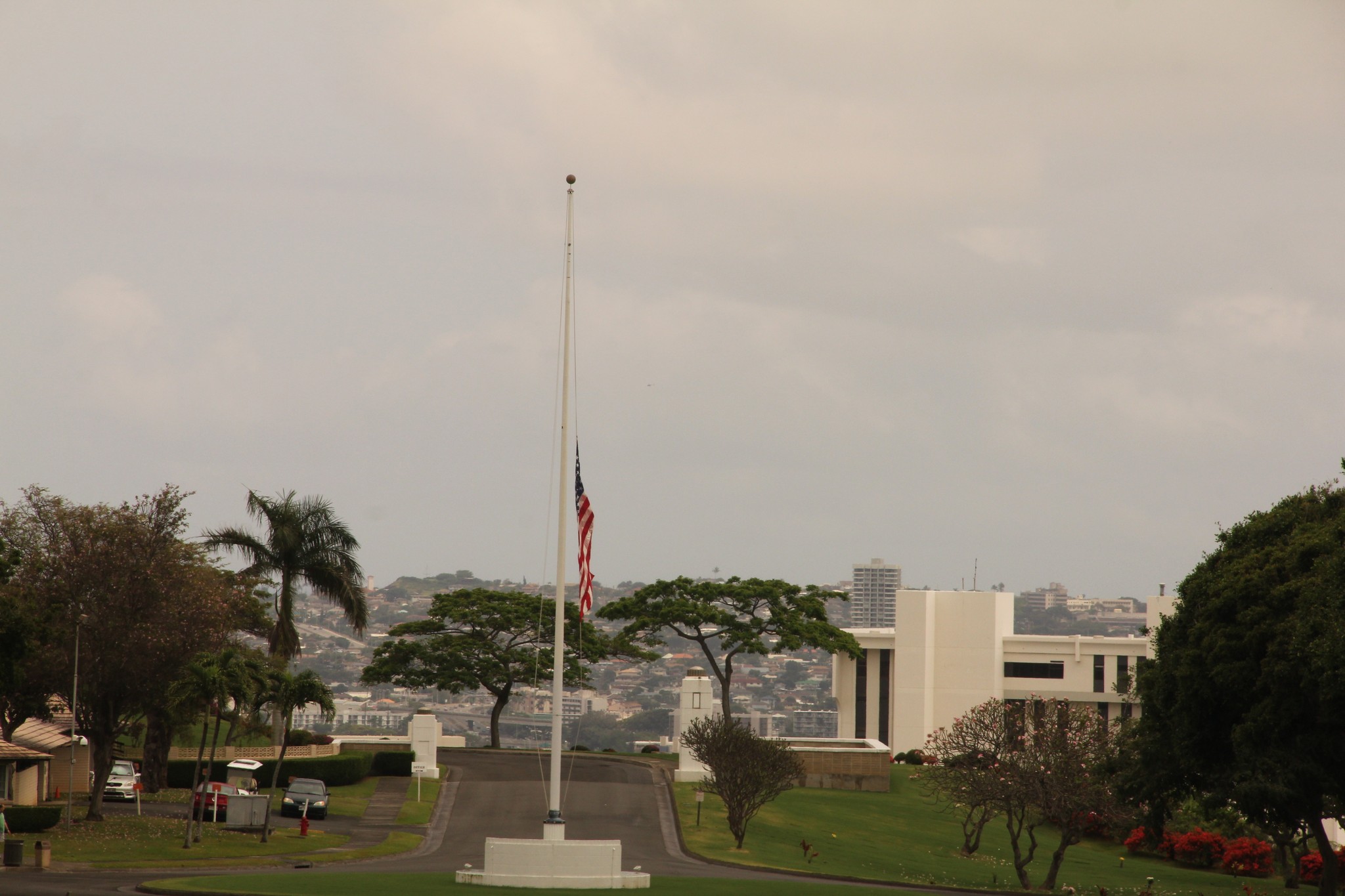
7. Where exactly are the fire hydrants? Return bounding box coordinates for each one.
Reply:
[298,816,309,835]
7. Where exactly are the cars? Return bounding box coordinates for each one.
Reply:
[281,778,331,820]
[89,760,141,802]
[193,782,239,816]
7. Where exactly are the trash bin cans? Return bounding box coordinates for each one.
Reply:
[35,840,52,868]
[3,839,26,867]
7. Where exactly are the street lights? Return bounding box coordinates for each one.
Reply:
[66,604,88,834]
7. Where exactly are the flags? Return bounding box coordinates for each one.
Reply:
[575,443,596,621]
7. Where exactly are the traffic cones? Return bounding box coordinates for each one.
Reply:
[54,785,60,798]
